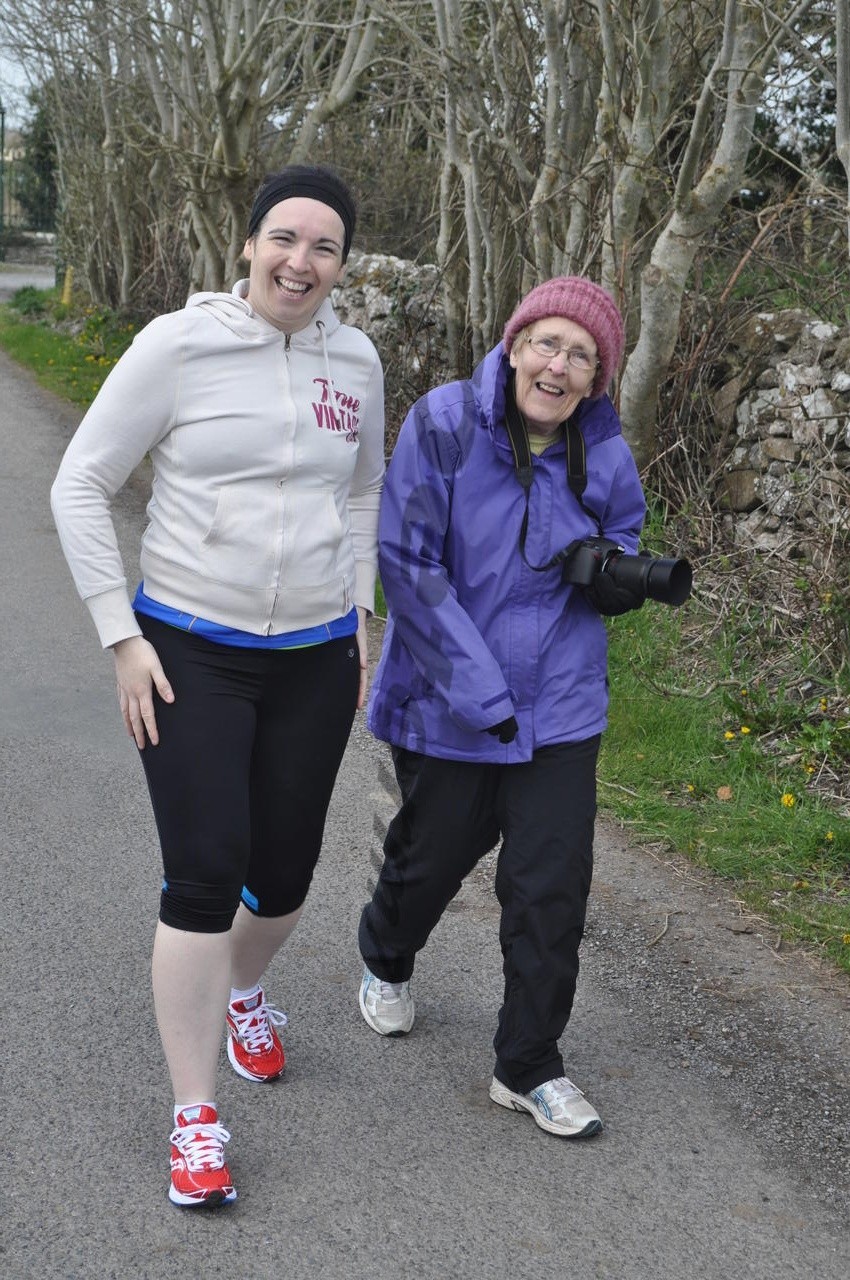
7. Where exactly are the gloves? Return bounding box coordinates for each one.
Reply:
[479,714,520,744]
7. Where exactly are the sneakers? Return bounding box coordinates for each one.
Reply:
[359,961,415,1037]
[168,1105,237,1206]
[226,984,287,1083]
[489,1075,603,1138]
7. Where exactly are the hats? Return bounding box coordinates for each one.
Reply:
[503,278,626,399]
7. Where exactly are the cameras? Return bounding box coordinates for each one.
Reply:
[560,536,694,610]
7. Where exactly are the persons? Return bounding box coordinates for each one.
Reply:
[357,277,646,1137]
[51,164,384,1208]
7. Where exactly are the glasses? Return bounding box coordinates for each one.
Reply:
[523,327,602,370]
